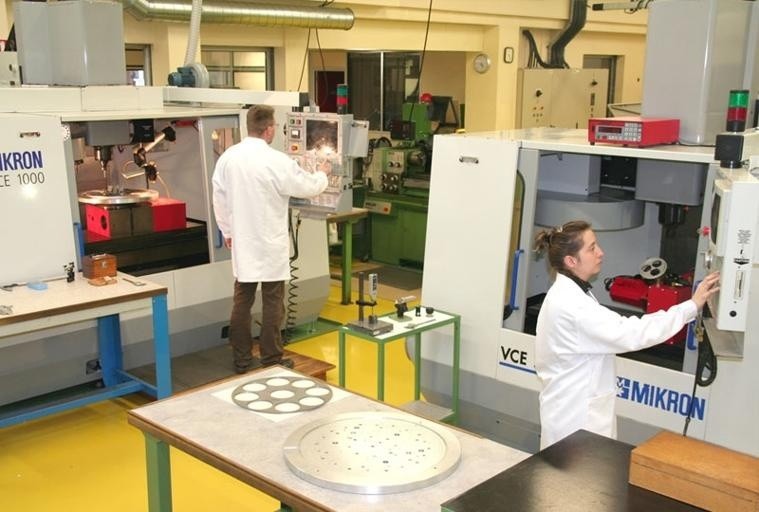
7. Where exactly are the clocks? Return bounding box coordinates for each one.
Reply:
[502,45,516,63]
[472,50,491,74]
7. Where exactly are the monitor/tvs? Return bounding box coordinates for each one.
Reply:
[709,180,732,257]
[303,117,352,160]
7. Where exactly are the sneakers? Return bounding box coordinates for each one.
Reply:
[235,363,248,374]
[281,358,294,370]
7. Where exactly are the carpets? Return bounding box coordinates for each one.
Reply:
[325,259,422,302]
[126,342,263,393]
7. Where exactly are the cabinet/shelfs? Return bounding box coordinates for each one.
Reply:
[334,306,462,433]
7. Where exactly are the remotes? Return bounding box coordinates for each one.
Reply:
[123,276,146,286]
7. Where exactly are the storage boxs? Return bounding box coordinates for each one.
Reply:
[628,427,759,512]
[82,253,119,279]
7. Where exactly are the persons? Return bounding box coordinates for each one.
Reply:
[211,105,333,374]
[531,220,721,451]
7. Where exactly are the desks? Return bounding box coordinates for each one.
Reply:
[124,361,534,512]
[329,205,370,309]
[440,425,706,512]
[0,271,174,432]
